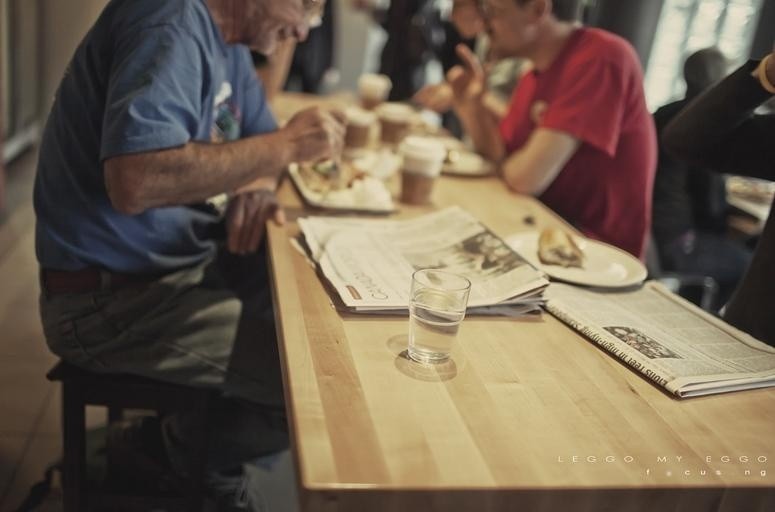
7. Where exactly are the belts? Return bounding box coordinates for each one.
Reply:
[40,264,177,295]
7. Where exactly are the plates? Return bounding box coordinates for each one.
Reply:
[503,230,649,289]
[440,149,495,177]
[286,160,394,213]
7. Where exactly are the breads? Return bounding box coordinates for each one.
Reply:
[435,137,484,169]
[538,222,586,270]
[298,157,371,194]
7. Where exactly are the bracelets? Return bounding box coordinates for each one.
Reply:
[758,53,775,96]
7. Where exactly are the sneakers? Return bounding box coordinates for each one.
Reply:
[109,414,260,511]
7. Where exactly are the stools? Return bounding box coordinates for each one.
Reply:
[44,356,289,511]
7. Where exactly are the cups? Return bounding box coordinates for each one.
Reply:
[408,268,471,366]
[399,135,444,206]
[341,73,413,161]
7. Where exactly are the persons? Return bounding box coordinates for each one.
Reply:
[659,50,775,353]
[351,1,657,267]
[651,42,753,316]
[28,1,351,511]
